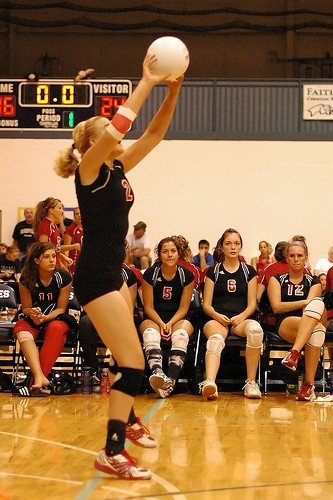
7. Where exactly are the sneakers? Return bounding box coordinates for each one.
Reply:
[242,379,262,399]
[95,447,151,480]
[296,381,316,401]
[125,417,157,449]
[148,368,174,398]
[198,378,218,400]
[281,350,300,371]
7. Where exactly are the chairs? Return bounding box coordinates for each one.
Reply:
[0,273,333,397]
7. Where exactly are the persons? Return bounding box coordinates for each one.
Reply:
[0,198,333,402]
[54,51,185,481]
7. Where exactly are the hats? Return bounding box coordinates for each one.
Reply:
[133,221,147,232]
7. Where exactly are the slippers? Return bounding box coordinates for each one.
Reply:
[12,386,50,397]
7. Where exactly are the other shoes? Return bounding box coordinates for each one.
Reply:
[81,360,100,394]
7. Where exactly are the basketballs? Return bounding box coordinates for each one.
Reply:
[147,37,189,79]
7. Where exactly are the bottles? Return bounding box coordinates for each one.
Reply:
[100,368,110,395]
